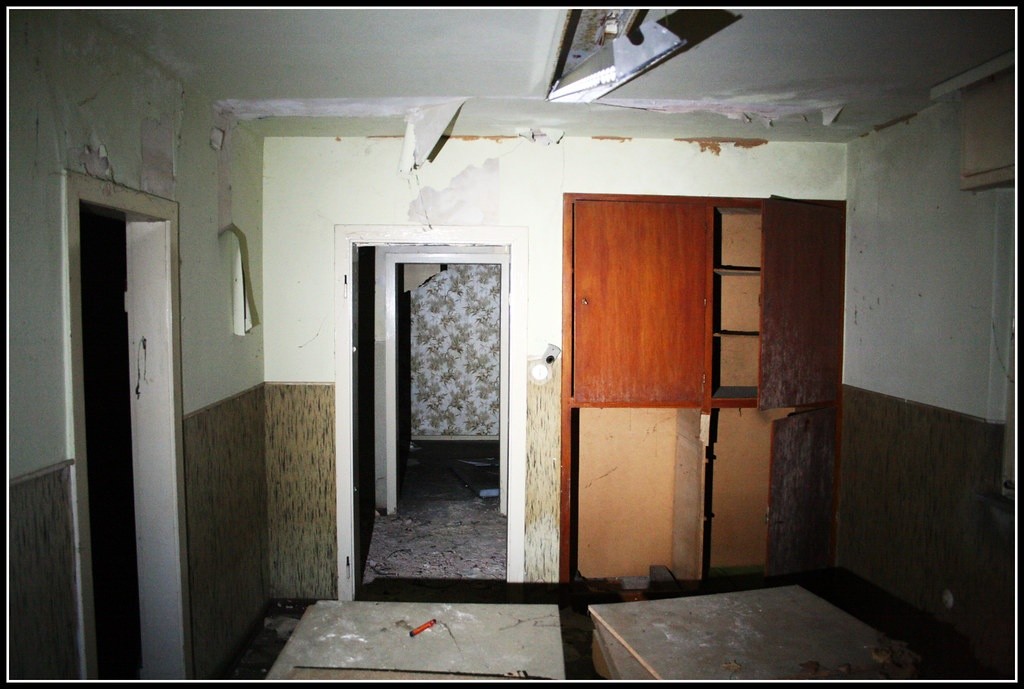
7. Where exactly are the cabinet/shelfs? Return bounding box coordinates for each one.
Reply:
[559,193,846,594]
